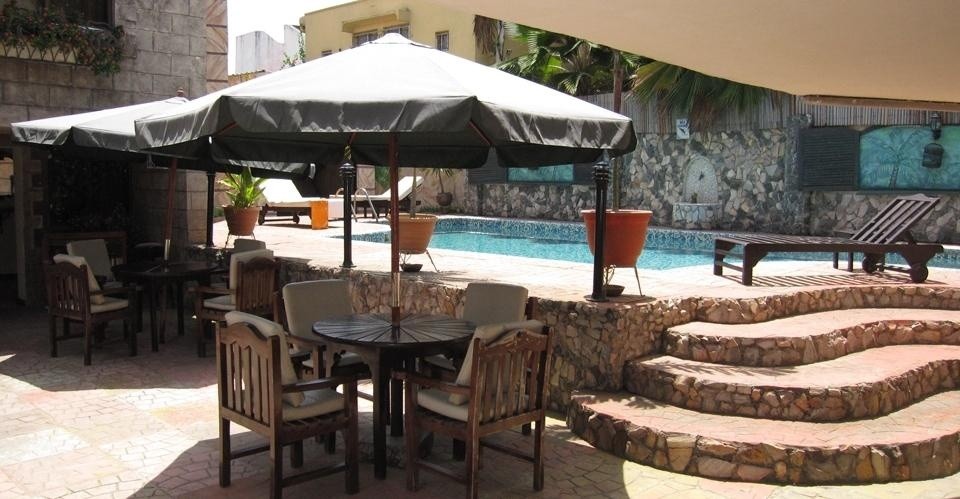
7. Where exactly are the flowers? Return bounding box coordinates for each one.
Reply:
[1,0,125,77]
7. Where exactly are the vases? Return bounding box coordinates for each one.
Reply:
[582,207,653,268]
[388,211,436,254]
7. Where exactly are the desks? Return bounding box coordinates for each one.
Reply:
[312,301,487,477]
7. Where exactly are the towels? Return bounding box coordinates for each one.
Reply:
[307,199,329,231]
[328,197,346,219]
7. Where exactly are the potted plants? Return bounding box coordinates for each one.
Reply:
[216,166,267,240]
[418,168,462,207]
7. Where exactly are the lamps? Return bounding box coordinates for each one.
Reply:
[928,110,945,140]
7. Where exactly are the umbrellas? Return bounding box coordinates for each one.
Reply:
[134,33,639,438]
[10,86,317,346]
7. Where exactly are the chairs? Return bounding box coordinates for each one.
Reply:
[389,311,562,498]
[208,306,365,498]
[330,174,427,223]
[39,235,283,370]
[424,280,533,456]
[709,188,949,289]
[280,276,379,443]
[246,172,350,227]
